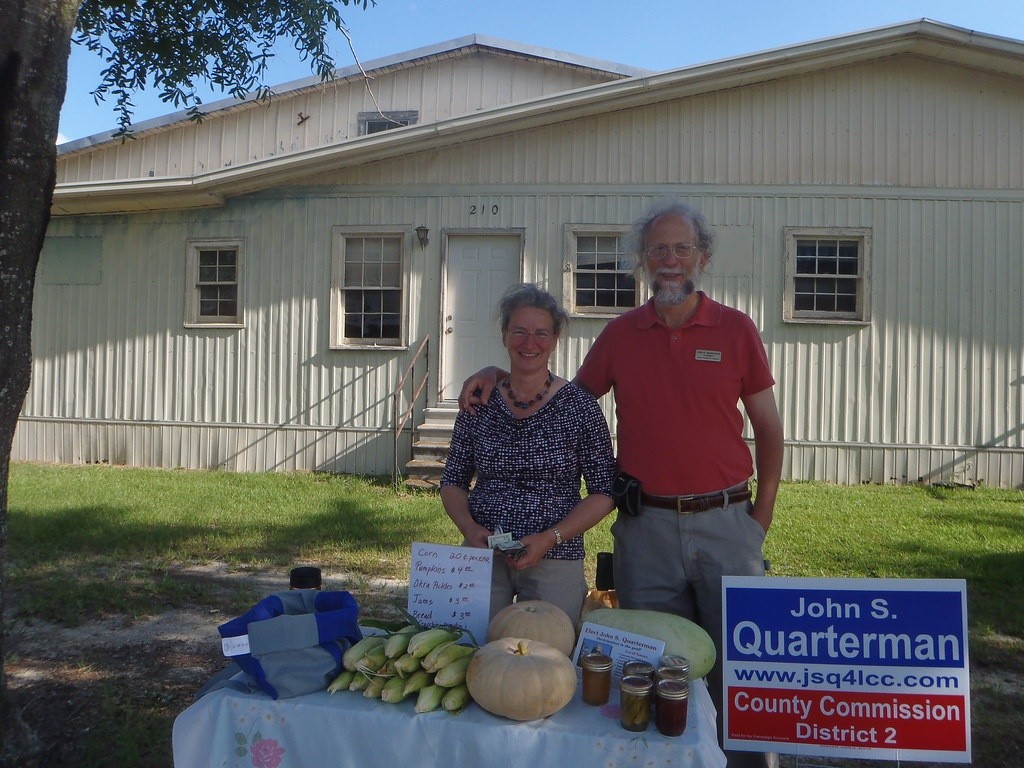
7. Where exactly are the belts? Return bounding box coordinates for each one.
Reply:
[641,484,752,514]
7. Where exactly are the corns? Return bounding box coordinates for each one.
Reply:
[326,626,481,713]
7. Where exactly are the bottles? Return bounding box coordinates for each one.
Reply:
[288,566,323,593]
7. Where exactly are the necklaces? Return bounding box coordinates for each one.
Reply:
[502,370,554,408]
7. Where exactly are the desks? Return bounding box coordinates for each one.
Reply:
[171,627,729,768]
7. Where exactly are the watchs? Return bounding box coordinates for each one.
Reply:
[551,525,562,547]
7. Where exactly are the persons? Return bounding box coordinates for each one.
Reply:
[440,283,617,630]
[458,204,784,768]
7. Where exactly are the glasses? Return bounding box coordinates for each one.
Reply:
[642,242,701,260]
[504,327,556,344]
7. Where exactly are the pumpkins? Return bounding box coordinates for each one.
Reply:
[485,600,575,657]
[466,637,577,721]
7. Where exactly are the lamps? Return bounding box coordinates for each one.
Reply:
[415,223,430,251]
